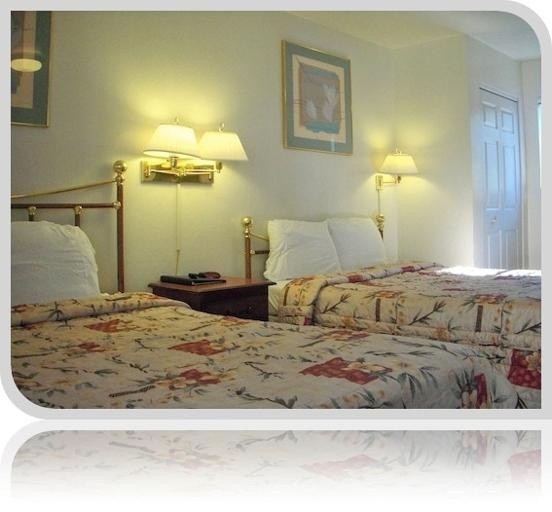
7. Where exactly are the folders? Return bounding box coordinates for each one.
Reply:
[160,275,227,286]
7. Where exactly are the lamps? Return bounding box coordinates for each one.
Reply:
[375,146,419,192]
[140,115,248,187]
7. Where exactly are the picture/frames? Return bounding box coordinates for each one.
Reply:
[11,11,54,128]
[280,38,353,156]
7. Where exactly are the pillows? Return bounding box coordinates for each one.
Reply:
[11,220,101,303]
[324,217,389,270]
[263,219,342,282]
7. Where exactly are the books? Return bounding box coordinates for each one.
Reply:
[160,274,226,287]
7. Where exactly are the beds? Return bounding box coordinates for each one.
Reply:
[11,159,527,409]
[242,213,541,409]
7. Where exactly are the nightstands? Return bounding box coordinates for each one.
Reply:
[145,276,276,322]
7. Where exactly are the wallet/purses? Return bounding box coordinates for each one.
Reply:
[189,272,221,278]
